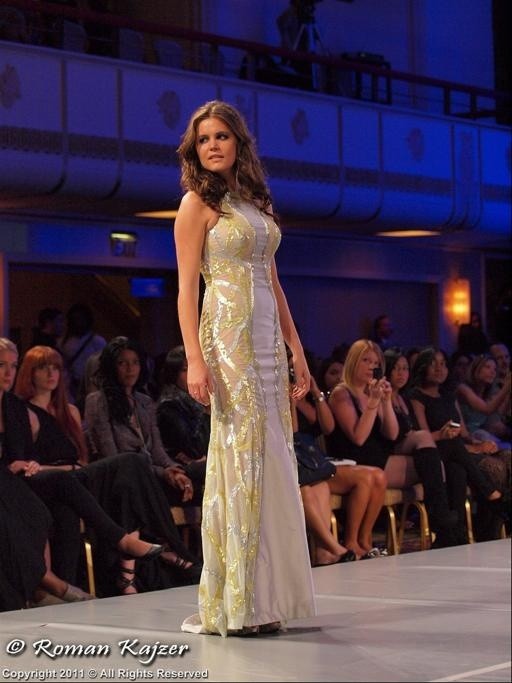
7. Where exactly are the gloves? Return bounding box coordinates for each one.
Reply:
[293,431,336,485]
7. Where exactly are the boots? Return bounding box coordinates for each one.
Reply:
[413,447,458,549]
[438,444,472,544]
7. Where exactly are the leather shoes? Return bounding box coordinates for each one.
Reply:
[39,592,68,605]
[260,621,280,634]
[122,542,165,560]
[233,626,260,636]
[63,583,96,601]
[314,550,355,566]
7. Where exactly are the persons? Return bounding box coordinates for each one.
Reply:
[41,0,114,57]
[173,99,317,638]
[277,0,318,89]
[0,303,512,609]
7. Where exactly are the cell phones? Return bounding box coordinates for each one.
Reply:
[373,368,382,380]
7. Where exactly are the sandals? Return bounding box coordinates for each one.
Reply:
[152,538,194,573]
[114,565,139,594]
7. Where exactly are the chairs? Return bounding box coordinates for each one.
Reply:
[164,431,474,555]
